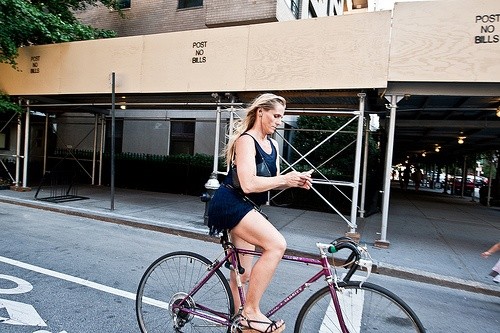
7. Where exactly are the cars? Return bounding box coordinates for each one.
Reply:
[452,174,489,194]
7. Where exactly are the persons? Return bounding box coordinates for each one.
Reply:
[481,241,500,283]
[208,93,314,333]
[402,164,423,192]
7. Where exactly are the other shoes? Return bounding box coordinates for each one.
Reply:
[493,275,500,282]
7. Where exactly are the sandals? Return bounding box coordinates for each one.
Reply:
[240,313,285,333]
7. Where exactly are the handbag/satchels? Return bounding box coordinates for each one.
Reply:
[230,133,272,188]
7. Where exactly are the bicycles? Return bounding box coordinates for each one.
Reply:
[135,227,426,333]
